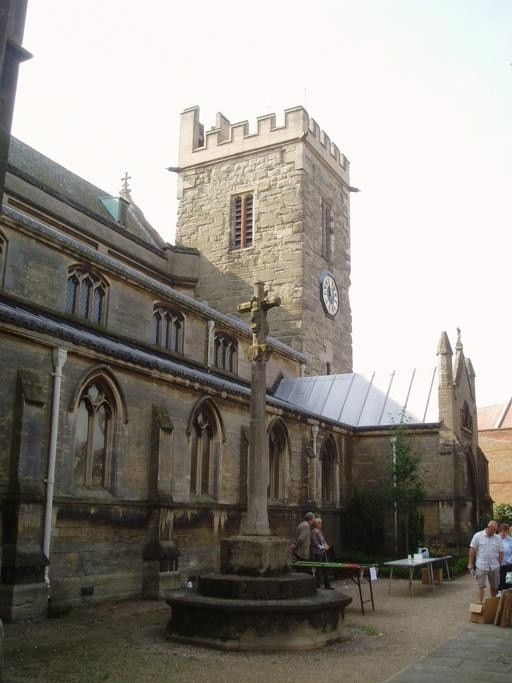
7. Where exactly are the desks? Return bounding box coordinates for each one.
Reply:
[292,560,380,616]
[384,555,453,597]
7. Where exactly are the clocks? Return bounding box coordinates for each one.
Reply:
[320,275,339,318]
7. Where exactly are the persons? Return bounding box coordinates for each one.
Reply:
[497,523,512,590]
[310,518,335,589]
[291,511,315,572]
[467,520,504,602]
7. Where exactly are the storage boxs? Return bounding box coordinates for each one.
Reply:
[421,568,442,585]
[470,601,486,624]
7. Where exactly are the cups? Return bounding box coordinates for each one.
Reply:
[408,554,411,559]
[187,581,192,587]
